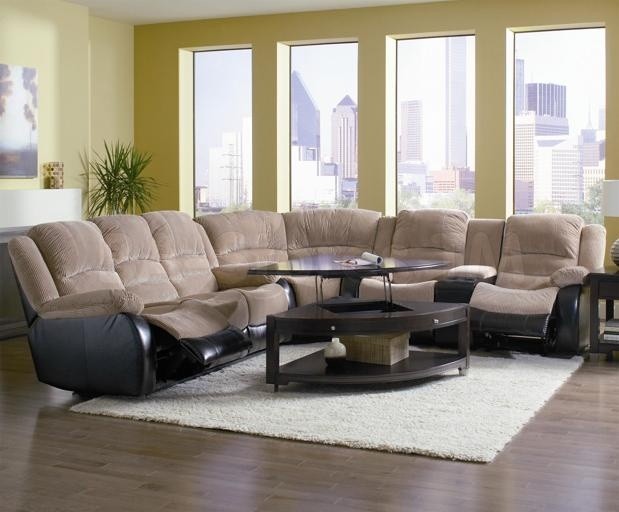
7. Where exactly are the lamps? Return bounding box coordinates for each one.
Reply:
[603,180,619,268]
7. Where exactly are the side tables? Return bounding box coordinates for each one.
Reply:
[587,268,618,362]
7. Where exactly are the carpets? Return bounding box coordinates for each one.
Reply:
[71,340,586,465]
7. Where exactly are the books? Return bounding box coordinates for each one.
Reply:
[603,321,618,341]
[333,252,383,267]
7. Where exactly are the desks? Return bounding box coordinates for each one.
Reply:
[0,188,82,342]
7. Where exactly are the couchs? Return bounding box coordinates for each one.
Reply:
[8,208,608,397]
